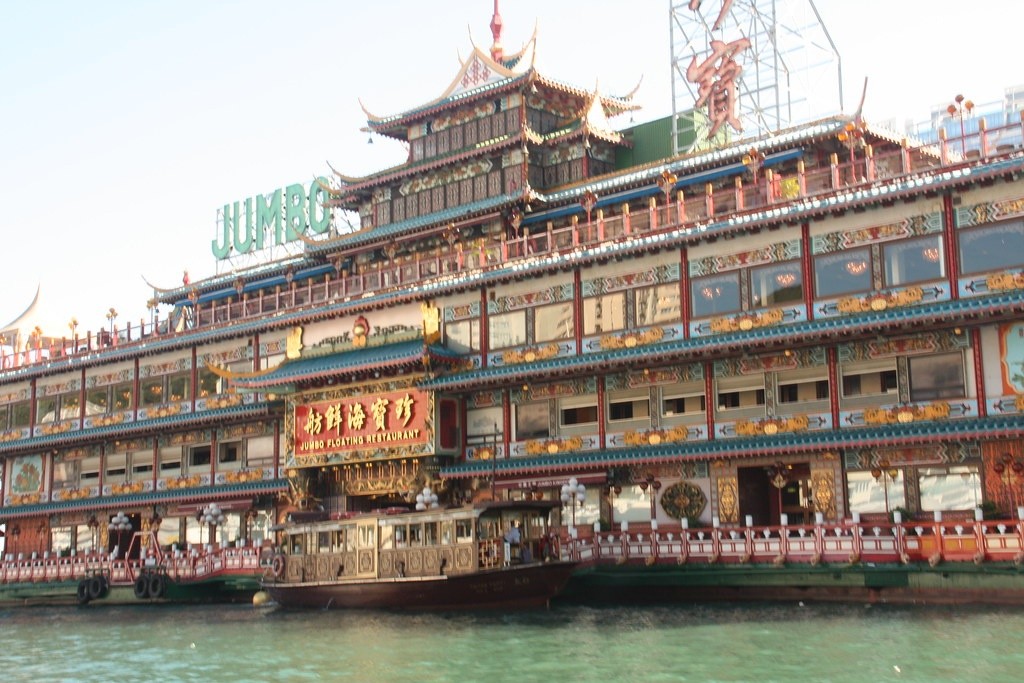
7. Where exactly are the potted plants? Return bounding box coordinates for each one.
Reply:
[973,499,1005,534]
[890,508,912,536]
[685,516,707,540]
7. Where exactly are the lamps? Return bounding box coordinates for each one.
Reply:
[921,239,939,264]
[845,258,867,275]
[699,286,724,301]
[775,270,798,288]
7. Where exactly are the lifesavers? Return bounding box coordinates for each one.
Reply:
[148,573,166,597]
[78,580,89,601]
[134,576,149,598]
[273,555,285,577]
[88,575,106,598]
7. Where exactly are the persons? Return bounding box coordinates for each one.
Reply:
[518,523,524,548]
[507,520,519,546]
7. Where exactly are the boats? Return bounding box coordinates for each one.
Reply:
[257,497,573,613]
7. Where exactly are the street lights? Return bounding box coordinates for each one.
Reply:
[640,473,661,517]
[872,456,898,535]
[994,453,1023,531]
[107,511,132,558]
[199,502,225,543]
[560,477,586,525]
[767,460,791,513]
